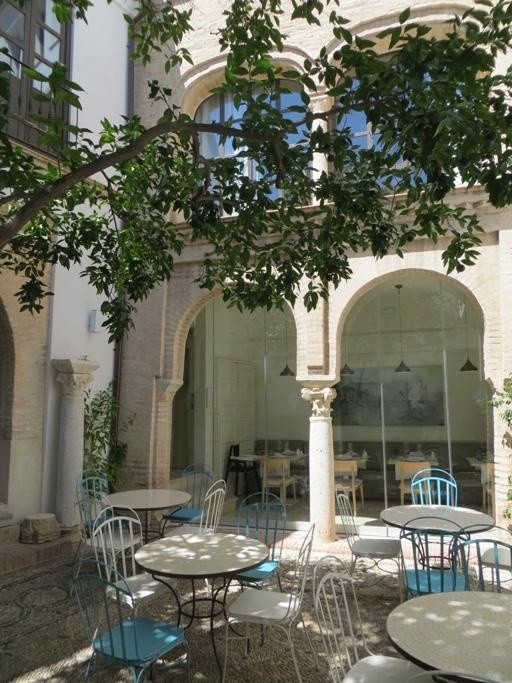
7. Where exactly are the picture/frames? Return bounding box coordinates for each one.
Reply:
[331,364,447,427]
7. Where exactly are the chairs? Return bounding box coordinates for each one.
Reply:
[69,444,512,682]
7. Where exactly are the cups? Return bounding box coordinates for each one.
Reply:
[269,440,291,457]
[347,441,354,453]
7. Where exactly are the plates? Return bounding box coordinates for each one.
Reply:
[337,454,352,460]
[239,455,261,462]
[406,456,424,462]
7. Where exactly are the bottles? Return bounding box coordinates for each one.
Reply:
[430,451,436,461]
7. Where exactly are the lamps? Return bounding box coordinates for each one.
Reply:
[460,303,478,373]
[340,320,355,376]
[88,308,103,333]
[393,282,411,372]
[280,300,295,378]
